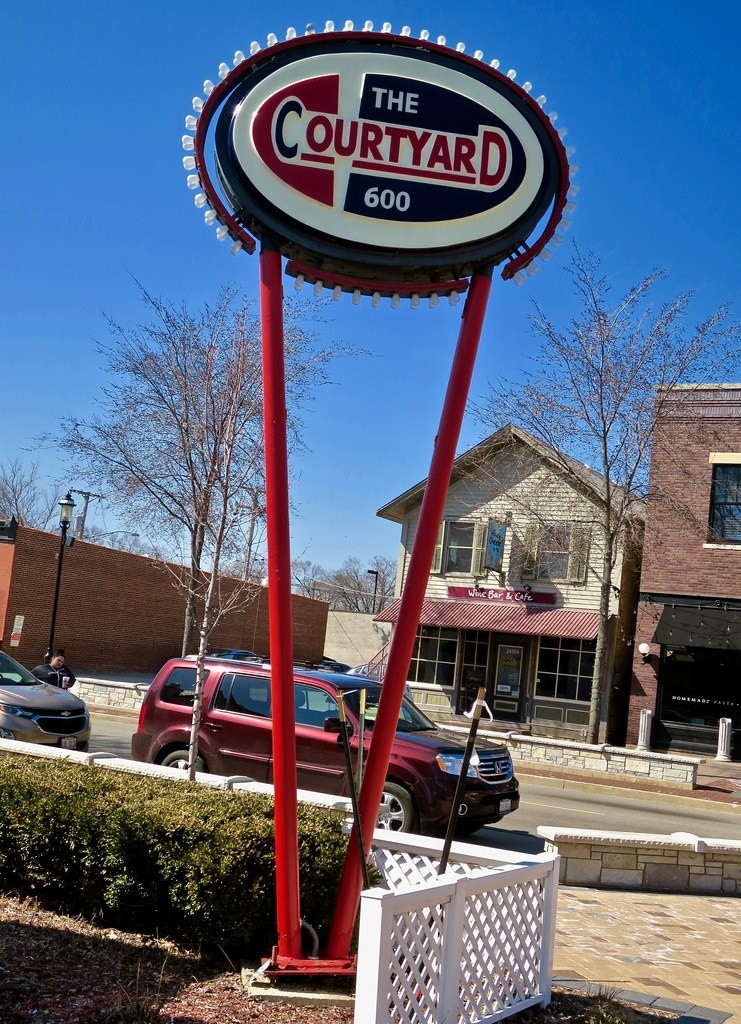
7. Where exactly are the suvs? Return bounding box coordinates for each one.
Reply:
[130,653,519,836]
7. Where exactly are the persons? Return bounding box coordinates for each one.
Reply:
[30,648,77,691]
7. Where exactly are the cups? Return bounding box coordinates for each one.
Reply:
[61,677,70,689]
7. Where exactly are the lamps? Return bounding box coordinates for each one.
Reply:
[639,643,651,665]
[522,584,532,590]
[474,578,480,590]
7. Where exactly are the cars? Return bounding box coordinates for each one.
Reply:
[0,647,90,752]
[203,647,387,678]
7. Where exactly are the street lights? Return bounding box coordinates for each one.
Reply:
[44,491,76,664]
[368,569,379,614]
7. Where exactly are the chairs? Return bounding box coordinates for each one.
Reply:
[295,689,306,709]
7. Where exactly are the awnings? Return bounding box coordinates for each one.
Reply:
[373,599,614,638]
[652,605,741,649]
